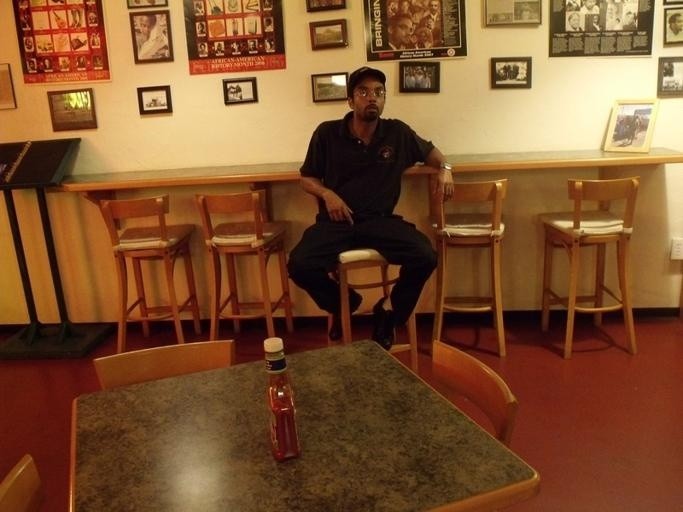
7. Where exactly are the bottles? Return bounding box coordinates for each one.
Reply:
[262,335,302,462]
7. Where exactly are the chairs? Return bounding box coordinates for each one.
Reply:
[331,238,420,376]
[420,335,519,449]
[527,172,644,363]
[421,171,511,358]
[0,450,46,512]
[185,185,297,344]
[96,190,202,354]
[87,335,238,392]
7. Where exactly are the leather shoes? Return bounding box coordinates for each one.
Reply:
[328,287,362,340]
[373,296,396,349]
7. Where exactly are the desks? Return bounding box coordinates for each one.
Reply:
[62,333,542,512]
[56,147,683,336]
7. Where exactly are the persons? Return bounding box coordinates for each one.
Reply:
[387,0,440,50]
[17,0,104,72]
[134,15,168,61]
[196,2,276,57]
[405,66,432,88]
[666,13,683,42]
[566,1,640,33]
[286,66,454,351]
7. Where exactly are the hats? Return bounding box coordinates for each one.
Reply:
[348,66,386,97]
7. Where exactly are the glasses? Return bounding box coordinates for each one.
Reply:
[354,89,384,97]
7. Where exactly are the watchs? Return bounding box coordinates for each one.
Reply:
[440,160,452,170]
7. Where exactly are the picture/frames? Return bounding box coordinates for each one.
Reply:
[304,0,353,104]
[661,7,682,46]
[488,55,531,91]
[661,0,682,6]
[395,59,440,94]
[655,54,682,99]
[482,0,543,28]
[0,0,259,137]
[360,0,469,63]
[600,97,662,155]
